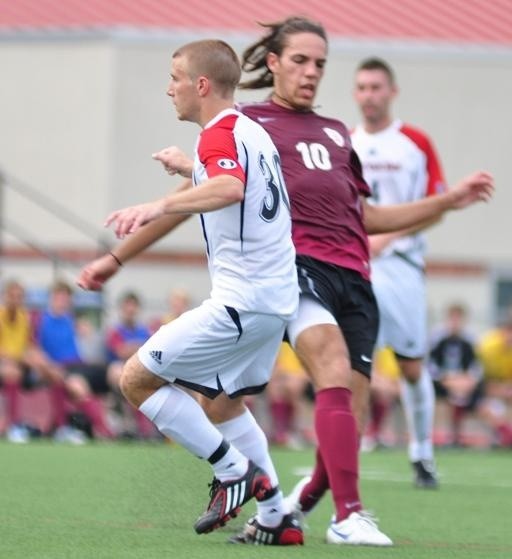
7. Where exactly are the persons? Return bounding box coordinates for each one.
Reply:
[358,302,511,454]
[266,341,307,452]
[101,39,304,547]
[0,279,193,445]
[340,57,453,492]
[76,13,497,548]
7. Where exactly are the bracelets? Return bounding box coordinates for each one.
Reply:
[109,251,124,267]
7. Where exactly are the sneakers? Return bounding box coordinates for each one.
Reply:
[327,512,392,545]
[414,461,437,489]
[194,460,312,545]
